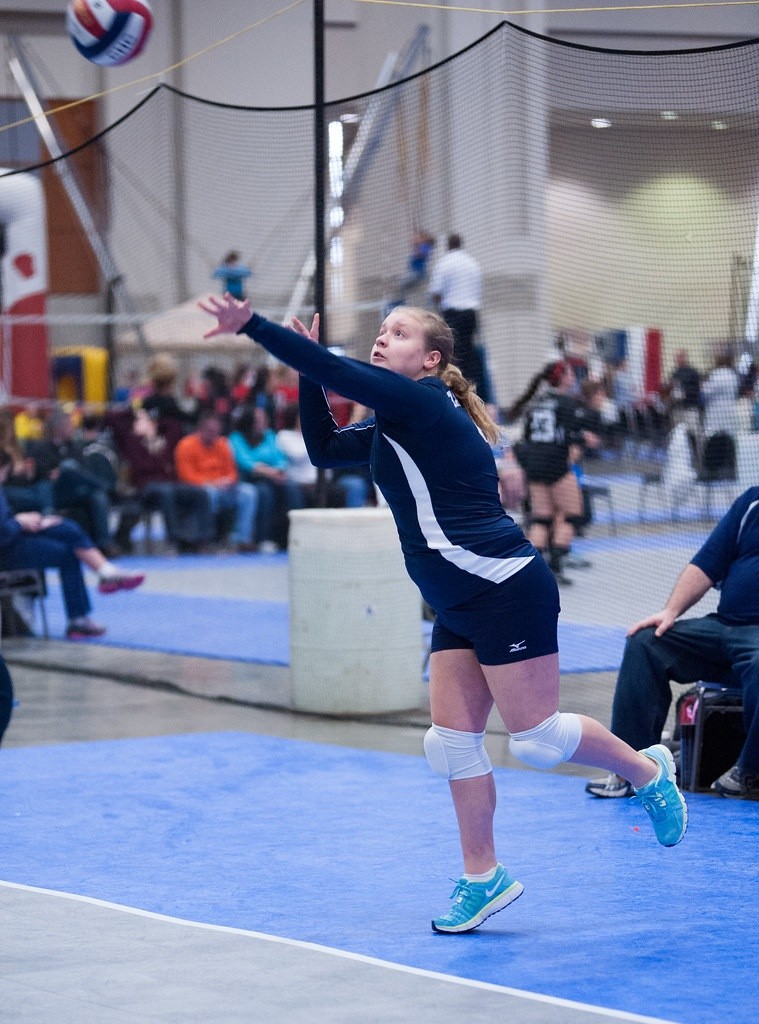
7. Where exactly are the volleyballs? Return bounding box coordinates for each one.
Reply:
[60,0,156,69]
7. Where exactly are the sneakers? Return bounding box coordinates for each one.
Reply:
[631,743,691,847]
[710,763,759,798]
[586,769,635,798]
[431,863,525,933]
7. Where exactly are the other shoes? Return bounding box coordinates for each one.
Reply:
[99,568,145,593]
[66,621,104,640]
[550,568,574,585]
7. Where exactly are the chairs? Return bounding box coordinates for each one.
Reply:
[669,429,743,521]
[0,569,51,640]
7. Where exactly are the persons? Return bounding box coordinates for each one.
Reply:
[199,290,689,936]
[1,232,758,642]
[584,486,758,800]
